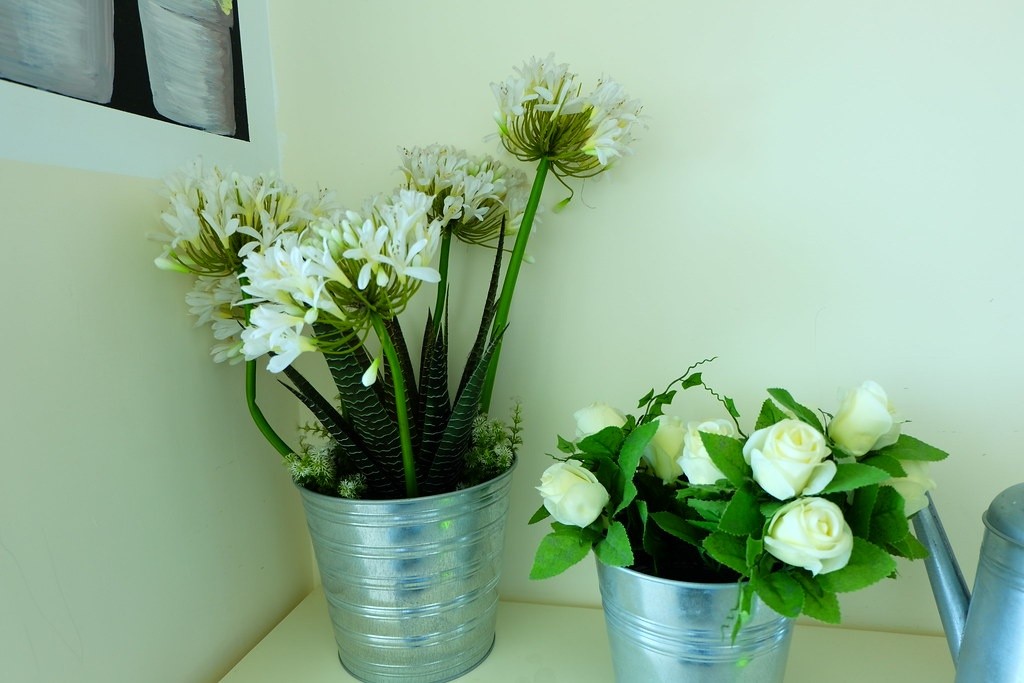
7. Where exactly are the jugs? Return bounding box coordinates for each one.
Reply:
[908,482,1024,683]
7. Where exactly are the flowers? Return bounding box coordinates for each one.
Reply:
[145,48,649,500]
[527,354,949,651]
